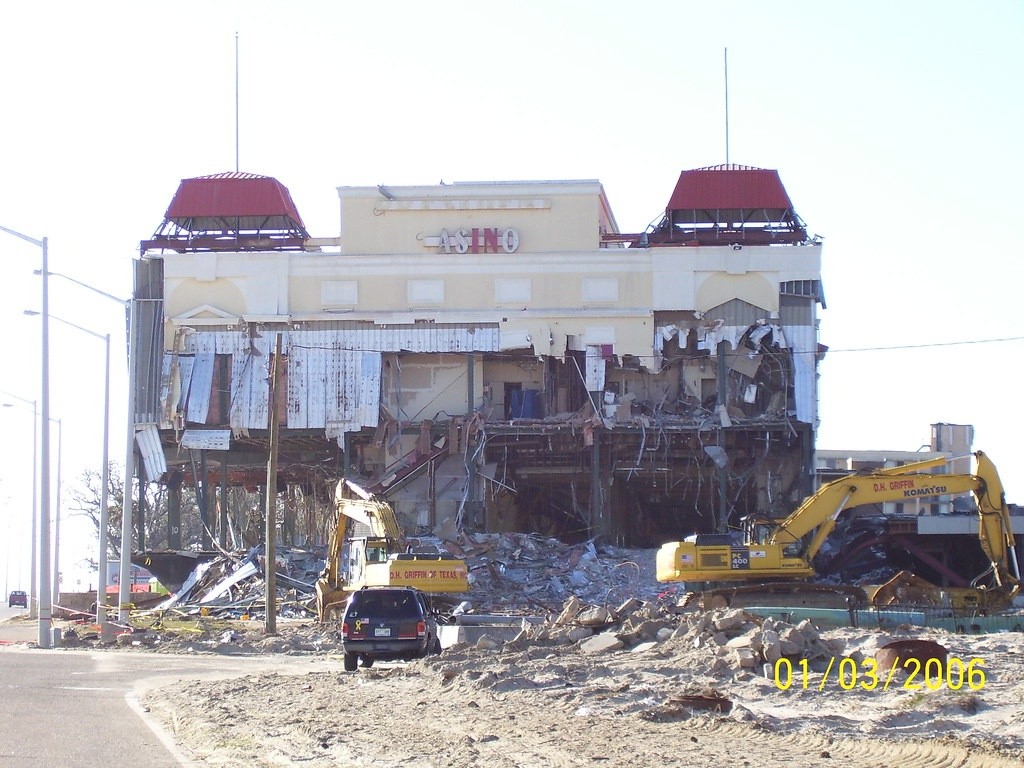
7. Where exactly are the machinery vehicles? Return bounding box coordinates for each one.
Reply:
[314,476,469,624]
[653,448,1024,615]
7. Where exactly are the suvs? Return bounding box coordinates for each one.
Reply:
[340,583,443,672]
[9,590,27,608]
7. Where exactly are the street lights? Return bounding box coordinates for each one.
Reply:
[22,308,110,623]
[32,268,139,624]
[4,402,63,614]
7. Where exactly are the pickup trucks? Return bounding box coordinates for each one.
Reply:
[9,591,28,608]
